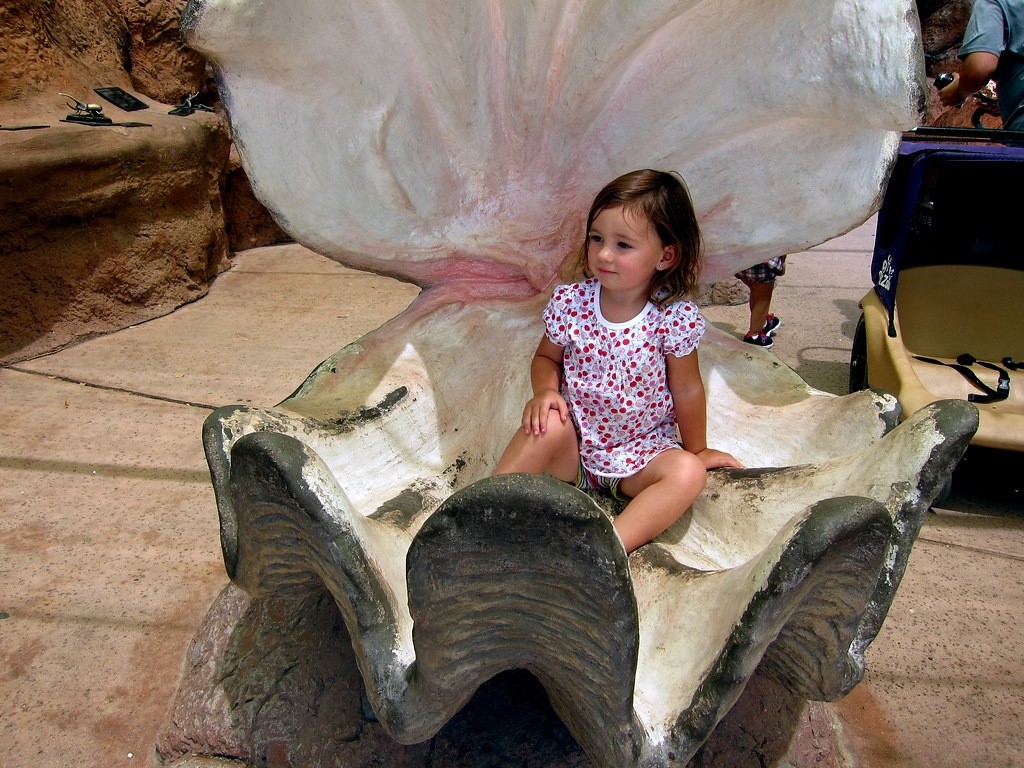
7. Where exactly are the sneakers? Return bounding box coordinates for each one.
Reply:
[743,331,774,348]
[762,313,780,337]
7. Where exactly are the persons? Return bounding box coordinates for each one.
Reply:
[735,255,788,348]
[493,169,747,554]
[938,0,1024,131]
